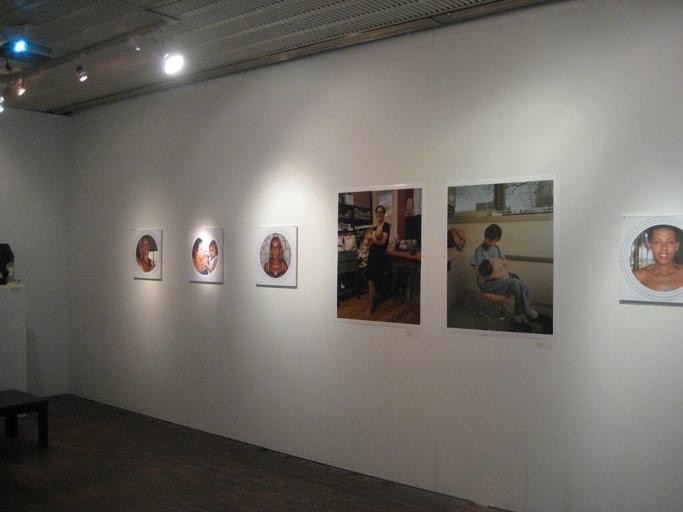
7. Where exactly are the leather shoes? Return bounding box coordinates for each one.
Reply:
[527,314,550,323]
[510,320,532,331]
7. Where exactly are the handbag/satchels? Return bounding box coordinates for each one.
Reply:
[448,229,465,247]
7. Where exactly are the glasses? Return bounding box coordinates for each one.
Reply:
[271,247,280,251]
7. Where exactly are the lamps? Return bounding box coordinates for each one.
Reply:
[152,42,186,76]
[75,64,88,83]
[15,80,27,98]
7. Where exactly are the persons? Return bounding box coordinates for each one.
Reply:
[479,258,509,278]
[471,224,548,331]
[137,238,155,273]
[362,231,384,254]
[192,239,208,275]
[209,241,218,272]
[633,226,683,291]
[360,205,392,319]
[264,237,288,278]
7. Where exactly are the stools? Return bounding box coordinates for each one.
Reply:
[0,388,51,446]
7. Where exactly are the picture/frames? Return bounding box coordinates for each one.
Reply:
[337,182,425,326]
[256,226,297,288]
[187,225,224,284]
[130,226,163,281]
[617,214,683,303]
[442,176,558,338]
